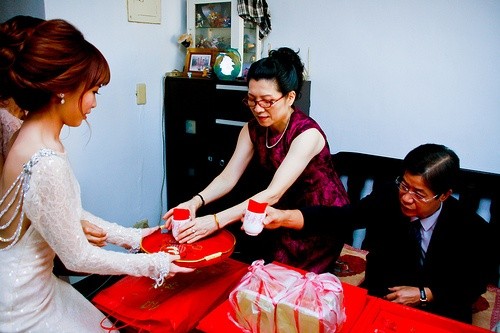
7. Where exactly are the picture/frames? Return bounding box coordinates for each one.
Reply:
[183,47,216,78]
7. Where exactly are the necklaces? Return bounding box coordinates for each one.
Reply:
[265,112,289,148]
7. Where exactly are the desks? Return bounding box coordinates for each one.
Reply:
[71,253,499,333]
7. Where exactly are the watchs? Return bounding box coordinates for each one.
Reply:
[419,286,428,306]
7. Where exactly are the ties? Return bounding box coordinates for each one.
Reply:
[409,219,422,275]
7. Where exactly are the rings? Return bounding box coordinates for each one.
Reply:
[191,226,195,232]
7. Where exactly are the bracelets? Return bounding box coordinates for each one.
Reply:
[213,213,220,230]
[196,193,205,207]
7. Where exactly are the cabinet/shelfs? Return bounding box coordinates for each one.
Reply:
[186,0,260,78]
[164,75,311,263]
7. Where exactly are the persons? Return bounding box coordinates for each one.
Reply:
[0,18,197,333]
[197,57,205,69]
[161,46,350,274]
[0,15,108,247]
[241,143,499,321]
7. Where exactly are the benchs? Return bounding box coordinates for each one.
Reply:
[332,151,500,333]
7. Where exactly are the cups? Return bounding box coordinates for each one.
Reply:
[243,209,267,236]
[172,217,191,240]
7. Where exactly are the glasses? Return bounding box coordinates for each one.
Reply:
[395,175,441,204]
[243,95,284,108]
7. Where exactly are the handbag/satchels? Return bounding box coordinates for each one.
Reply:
[92,257,248,333]
